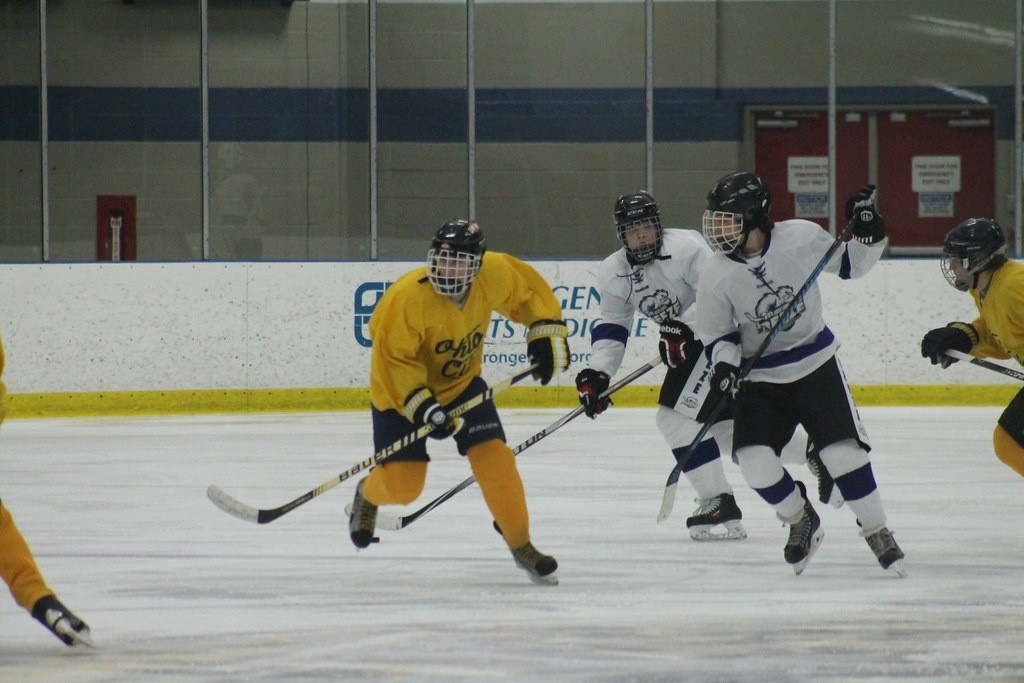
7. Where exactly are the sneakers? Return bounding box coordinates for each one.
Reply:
[32,596,100,651]
[512,542,559,585]
[686,493,747,540]
[856,517,906,577]
[806,443,845,507]
[349,477,379,550]
[775,481,825,576]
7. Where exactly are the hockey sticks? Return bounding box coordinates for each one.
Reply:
[655,210,860,524]
[343,355,663,531]
[207,362,540,526]
[944,348,1024,382]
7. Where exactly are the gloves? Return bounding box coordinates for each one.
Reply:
[659,319,701,378]
[404,385,456,440]
[921,321,980,369]
[527,319,572,385]
[845,184,886,244]
[709,362,747,399]
[575,368,610,419]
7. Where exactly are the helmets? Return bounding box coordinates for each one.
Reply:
[702,171,771,255]
[614,189,662,262]
[940,216,1007,291]
[426,219,488,297]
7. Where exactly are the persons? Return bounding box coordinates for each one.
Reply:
[702,172,907,579]
[0,337,97,650]
[576,189,843,539]
[350,217,570,589]
[921,216,1023,474]
